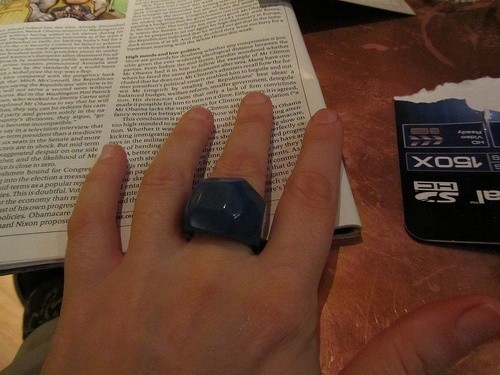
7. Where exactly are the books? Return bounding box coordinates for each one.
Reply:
[2,0,364,279]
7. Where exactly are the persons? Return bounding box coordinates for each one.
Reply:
[2,89,500,375]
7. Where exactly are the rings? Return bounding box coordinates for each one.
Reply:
[188,176,272,256]
